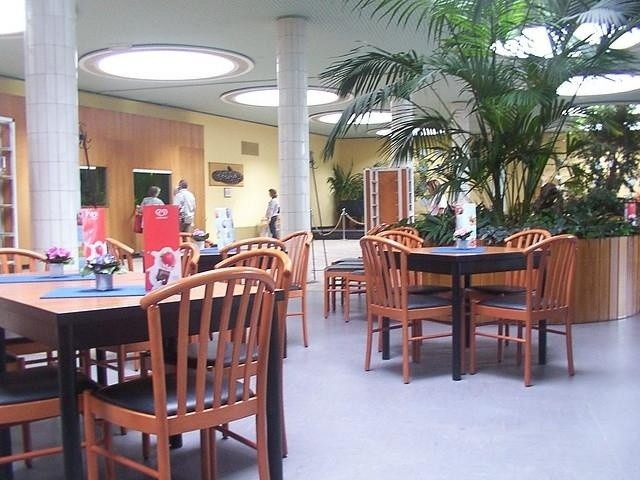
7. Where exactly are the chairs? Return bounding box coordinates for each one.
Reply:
[0,366,114,480]
[463,228,551,366]
[219,237,288,266]
[279,229,313,348]
[0,248,58,368]
[374,231,455,364]
[358,235,465,384]
[465,234,577,387]
[323,224,424,322]
[85,266,270,479]
[106,237,135,271]
[164,249,289,458]
[179,244,200,278]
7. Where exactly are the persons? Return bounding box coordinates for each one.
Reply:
[426,178,447,215]
[138,184,166,230]
[173,178,196,233]
[265,188,281,239]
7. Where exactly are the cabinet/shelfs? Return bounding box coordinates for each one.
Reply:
[0,116,20,266]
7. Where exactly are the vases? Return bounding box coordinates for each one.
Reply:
[95,273,112,290]
[50,264,63,278]
[456,240,466,251]
[195,242,204,250]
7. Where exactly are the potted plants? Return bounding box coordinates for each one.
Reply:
[315,0,640,327]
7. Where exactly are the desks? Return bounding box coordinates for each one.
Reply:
[180,248,246,273]
[377,245,550,381]
[0,270,283,478]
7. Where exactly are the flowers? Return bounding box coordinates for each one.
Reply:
[453,229,473,239]
[192,230,210,241]
[86,254,117,274]
[46,246,72,264]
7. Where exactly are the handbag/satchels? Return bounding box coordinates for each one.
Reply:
[133,213,144,234]
